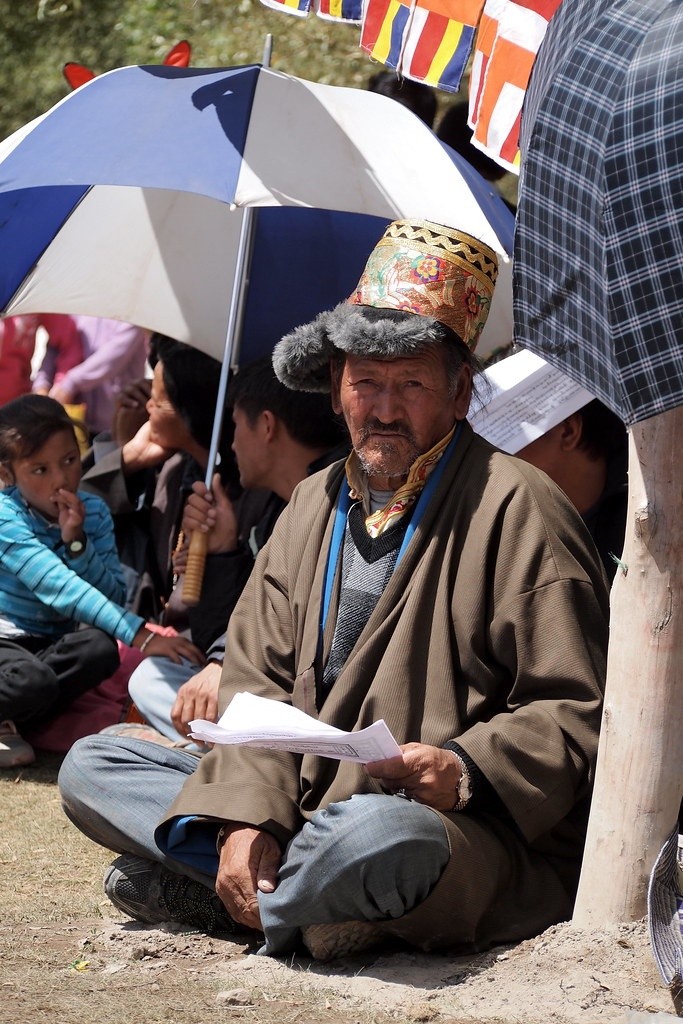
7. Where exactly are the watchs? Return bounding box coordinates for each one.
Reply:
[52,531,89,560]
[450,750,474,815]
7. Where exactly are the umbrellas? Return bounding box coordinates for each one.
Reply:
[0,0,682,604]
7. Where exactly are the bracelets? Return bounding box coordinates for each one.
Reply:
[139,632,159,653]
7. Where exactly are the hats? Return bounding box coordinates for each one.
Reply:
[272,217,497,392]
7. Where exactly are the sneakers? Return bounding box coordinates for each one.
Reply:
[300,921,387,962]
[103,853,245,935]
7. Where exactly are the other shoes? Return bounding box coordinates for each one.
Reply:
[0,718,35,768]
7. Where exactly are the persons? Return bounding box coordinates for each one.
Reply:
[0,220,624,956]
